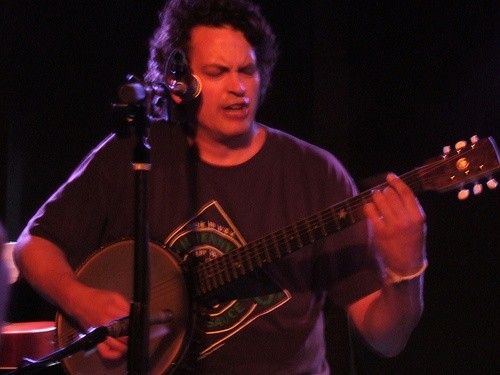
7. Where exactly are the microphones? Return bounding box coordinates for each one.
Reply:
[170,73,203,101]
[107,308,173,340]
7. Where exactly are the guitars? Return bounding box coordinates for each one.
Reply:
[51,132,500,374]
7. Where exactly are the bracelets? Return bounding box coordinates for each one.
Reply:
[383,259,428,286]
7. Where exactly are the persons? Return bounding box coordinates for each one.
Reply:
[11,0,429,372]
